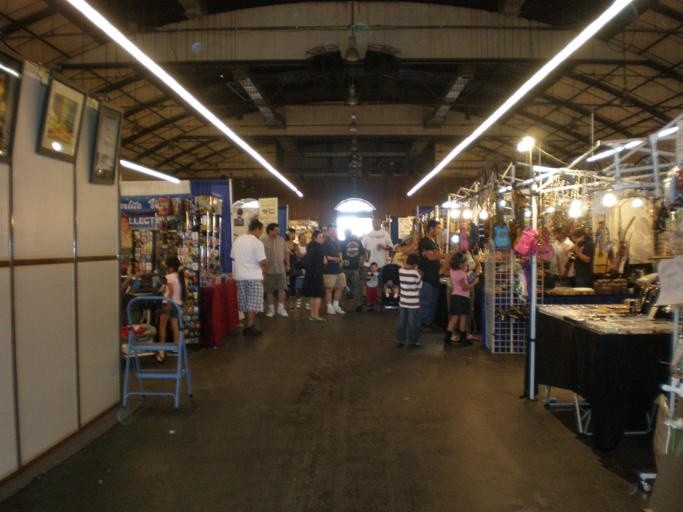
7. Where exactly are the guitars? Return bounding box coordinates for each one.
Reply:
[512,193,526,252]
[536,217,554,261]
[469,198,478,246]
[489,192,510,249]
[479,195,485,242]
[459,203,469,251]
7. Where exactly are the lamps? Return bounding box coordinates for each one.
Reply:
[344,1,364,183]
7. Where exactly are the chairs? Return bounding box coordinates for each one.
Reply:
[122,295,193,408]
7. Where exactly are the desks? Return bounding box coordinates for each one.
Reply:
[539,293,636,302]
[526,303,672,437]
[200,281,240,347]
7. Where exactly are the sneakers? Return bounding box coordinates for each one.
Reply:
[265,308,275,318]
[277,308,288,317]
[334,306,345,314]
[308,316,327,322]
[326,306,336,315]
[243,326,258,337]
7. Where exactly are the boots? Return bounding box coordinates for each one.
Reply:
[444,330,452,346]
[459,330,474,345]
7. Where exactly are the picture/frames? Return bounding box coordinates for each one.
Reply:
[0,52,24,164]
[36,71,88,164]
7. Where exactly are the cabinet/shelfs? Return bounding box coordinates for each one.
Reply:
[120,193,224,345]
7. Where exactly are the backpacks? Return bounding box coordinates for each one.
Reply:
[297,254,312,269]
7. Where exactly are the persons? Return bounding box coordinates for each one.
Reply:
[260,223,291,318]
[361,216,393,305]
[365,261,381,312]
[158,256,186,343]
[294,233,311,310]
[230,219,268,336]
[417,220,445,333]
[552,228,576,289]
[284,228,301,301]
[568,228,595,288]
[304,231,328,324]
[450,236,483,342]
[394,253,423,348]
[391,242,415,265]
[340,229,366,313]
[443,252,473,346]
[323,223,346,315]
